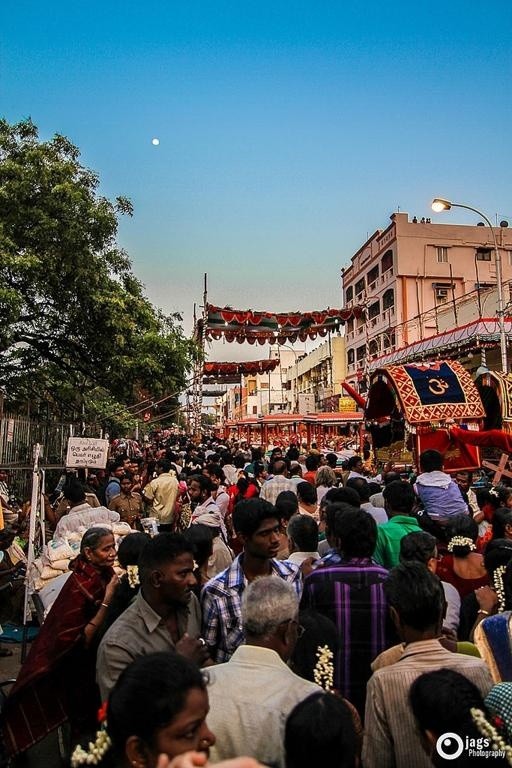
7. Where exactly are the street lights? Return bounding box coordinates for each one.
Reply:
[431,195,511,375]
[267,342,299,403]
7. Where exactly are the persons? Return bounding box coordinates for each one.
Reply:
[2,424,512,768]
